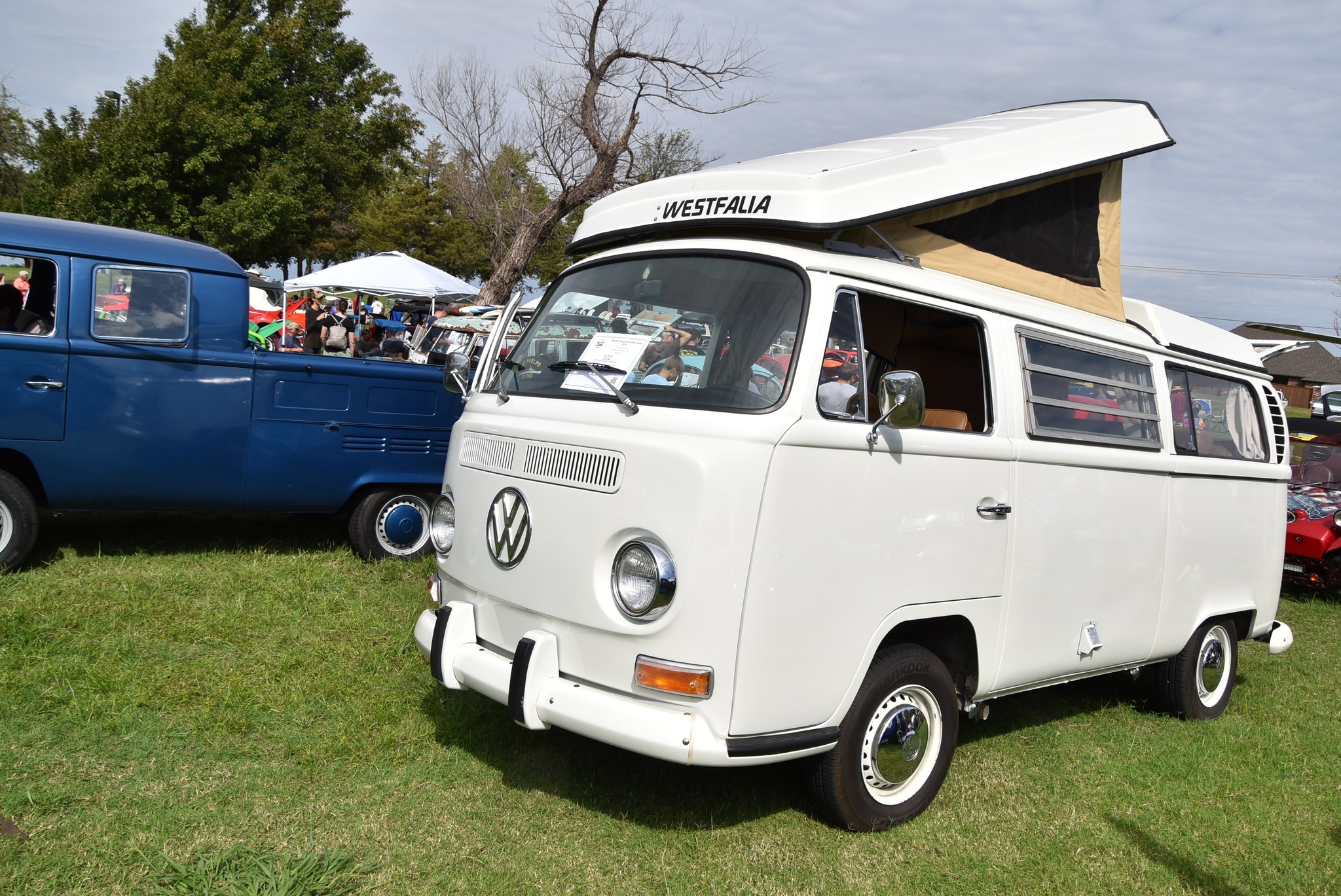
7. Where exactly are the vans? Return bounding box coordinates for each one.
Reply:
[413,99,1295,831]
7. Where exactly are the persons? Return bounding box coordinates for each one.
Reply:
[291,295,299,304]
[638,325,692,373]
[390,299,403,322]
[320,299,355,358]
[13,271,29,308]
[0,283,24,332]
[1093,382,1107,399]
[354,329,380,358]
[354,302,368,336]
[1170,386,1196,427]
[303,290,328,355]
[640,354,685,385]
[379,330,402,350]
[678,329,706,374]
[395,330,412,348]
[370,297,383,317]
[0,273,5,285]
[818,362,857,413]
[121,276,127,293]
[120,284,125,293]
[112,279,127,296]
[421,303,453,353]
[611,319,629,334]
[278,322,304,353]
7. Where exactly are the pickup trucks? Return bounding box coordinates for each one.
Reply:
[0,212,477,577]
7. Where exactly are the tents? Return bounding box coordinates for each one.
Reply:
[282,250,481,353]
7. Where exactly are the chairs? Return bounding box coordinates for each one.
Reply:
[1303,449,1341,490]
[24,320,54,335]
[1205,408,1230,432]
[381,340,404,357]
[1289,461,1337,490]
[14,308,53,331]
[921,409,972,432]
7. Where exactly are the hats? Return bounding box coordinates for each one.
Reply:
[1171,386,1184,391]
[310,290,326,299]
[361,329,369,335]
[288,322,301,329]
[361,302,365,306]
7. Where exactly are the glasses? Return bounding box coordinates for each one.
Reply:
[295,328,299,330]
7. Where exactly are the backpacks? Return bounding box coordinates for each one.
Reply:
[326,314,348,349]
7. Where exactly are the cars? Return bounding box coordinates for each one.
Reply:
[92,241,884,405]
[1276,390,1288,412]
[1281,436,1341,603]
[1310,396,1341,422]
